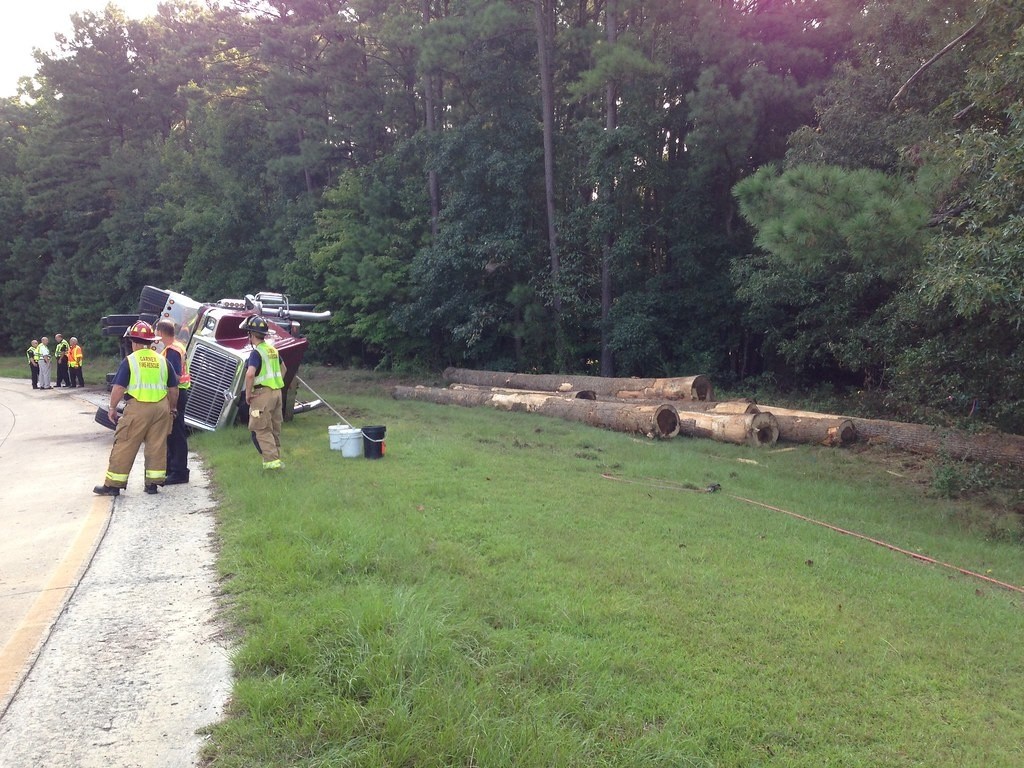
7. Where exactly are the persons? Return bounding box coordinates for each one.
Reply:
[68,337,85,388]
[26,337,53,389]
[239,314,287,471]
[53,333,70,387]
[93,320,180,496]
[156,320,191,486]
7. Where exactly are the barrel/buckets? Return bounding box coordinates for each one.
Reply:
[361,425,387,459]
[328,425,349,450]
[339,428,361,457]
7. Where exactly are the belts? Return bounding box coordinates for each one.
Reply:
[254,384,264,389]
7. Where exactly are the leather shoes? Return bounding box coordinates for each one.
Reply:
[92,485,121,496]
[144,485,158,494]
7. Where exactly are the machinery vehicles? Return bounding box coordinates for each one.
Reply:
[94,283,331,432]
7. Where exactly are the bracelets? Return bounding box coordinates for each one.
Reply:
[109,408,117,410]
[170,409,177,414]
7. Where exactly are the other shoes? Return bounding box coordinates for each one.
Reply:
[164,469,190,485]
[78,384,84,387]
[69,384,76,387]
[53,384,61,387]
[44,387,53,389]
[41,386,44,388]
[63,384,70,387]
[33,385,40,389]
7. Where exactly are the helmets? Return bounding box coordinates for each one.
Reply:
[122,320,163,345]
[239,314,271,335]
